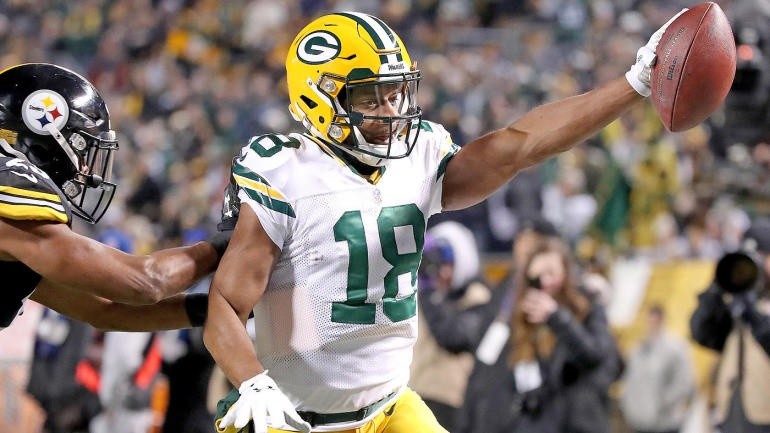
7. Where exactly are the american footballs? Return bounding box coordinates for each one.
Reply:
[651,2,737,134]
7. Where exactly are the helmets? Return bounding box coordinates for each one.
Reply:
[0,63,110,199]
[284,11,422,167]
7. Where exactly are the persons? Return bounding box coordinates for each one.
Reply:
[0,0,770,252]
[201,0,735,433]
[25,230,255,433]
[0,64,254,331]
[408,223,770,433]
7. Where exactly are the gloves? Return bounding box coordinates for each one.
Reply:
[218,374,311,433]
[625,7,691,97]
